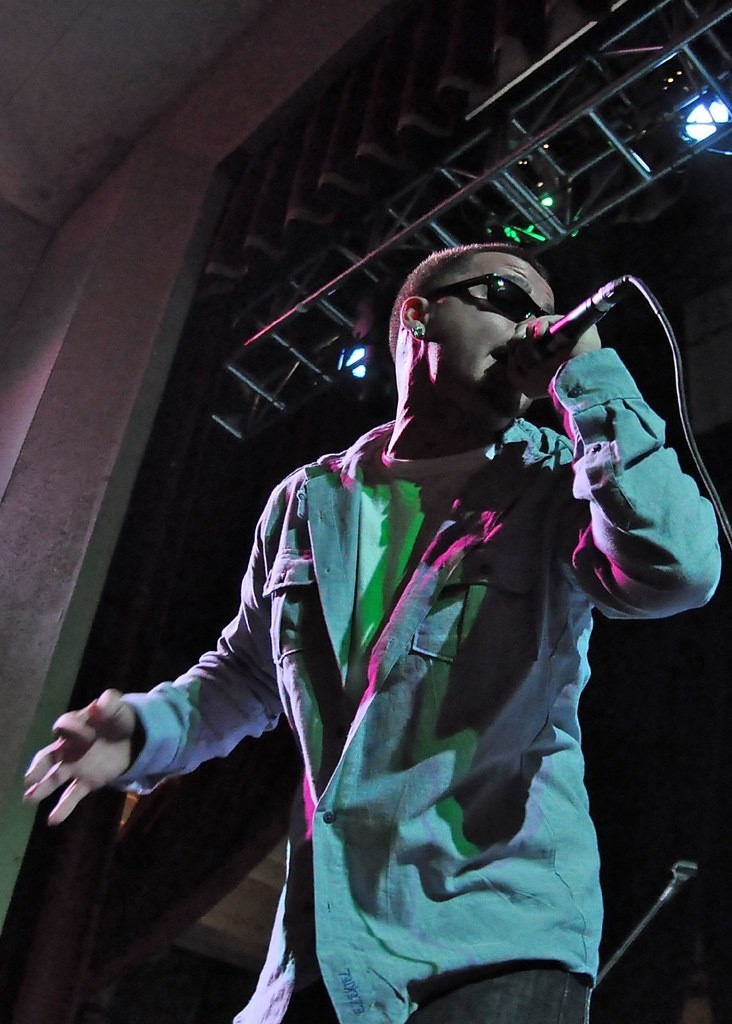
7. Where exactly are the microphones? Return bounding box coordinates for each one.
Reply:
[515,273,630,371]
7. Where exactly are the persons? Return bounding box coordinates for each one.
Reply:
[23,242,722,1024]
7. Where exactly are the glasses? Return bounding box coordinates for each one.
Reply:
[421,273,550,324]
